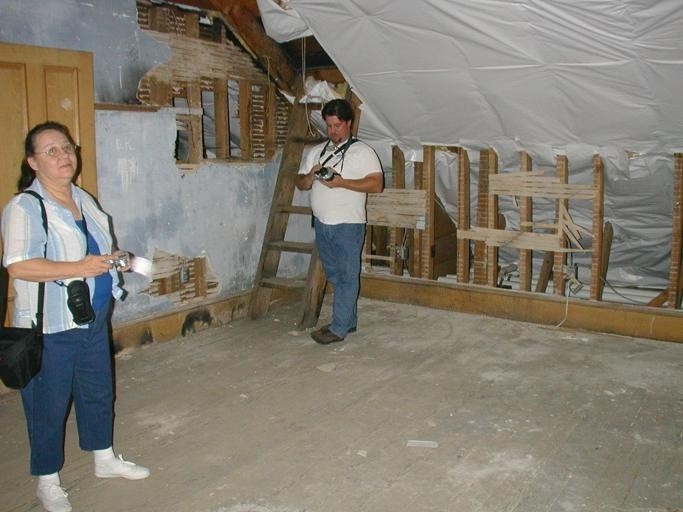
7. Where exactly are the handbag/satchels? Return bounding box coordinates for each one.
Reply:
[0,324,44,390]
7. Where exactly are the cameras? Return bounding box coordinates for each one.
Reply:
[314,166,341,181]
[103,253,128,269]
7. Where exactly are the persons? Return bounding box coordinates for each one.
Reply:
[0,123,151,512]
[295,99,384,344]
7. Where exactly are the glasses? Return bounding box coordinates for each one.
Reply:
[32,142,77,156]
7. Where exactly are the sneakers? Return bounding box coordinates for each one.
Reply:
[311,323,357,345]
[35,483,72,510]
[94,458,151,481]
